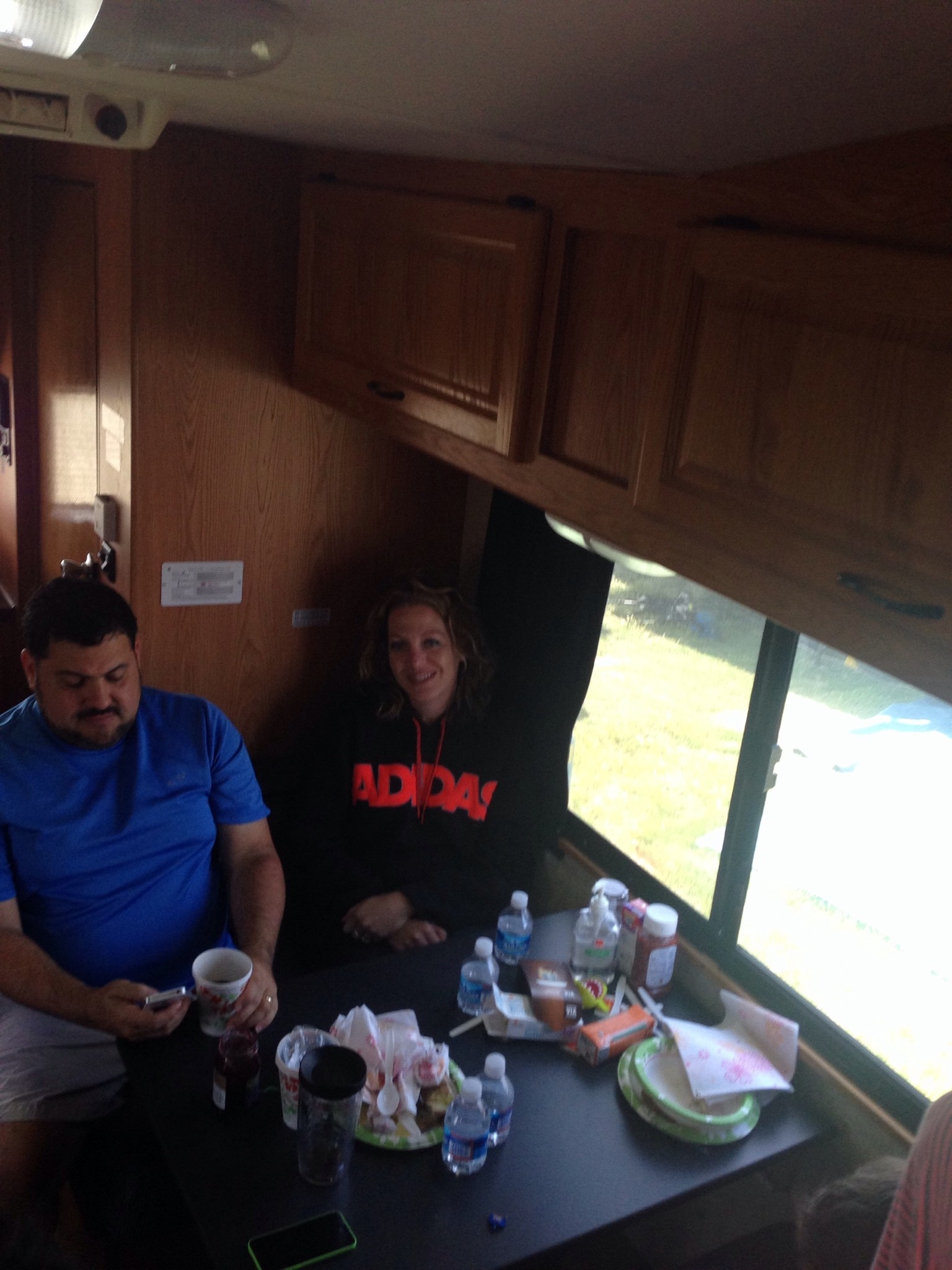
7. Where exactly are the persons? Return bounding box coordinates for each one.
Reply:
[304,584,578,967]
[793,1157,907,1270]
[0,579,287,1270]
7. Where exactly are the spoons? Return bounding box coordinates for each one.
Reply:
[377,1028,398,1114]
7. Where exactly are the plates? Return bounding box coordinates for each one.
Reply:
[617,1039,763,1145]
[357,1055,465,1150]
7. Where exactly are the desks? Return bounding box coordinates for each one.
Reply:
[110,896,844,1270]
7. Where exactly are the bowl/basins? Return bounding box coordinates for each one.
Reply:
[635,1034,754,1128]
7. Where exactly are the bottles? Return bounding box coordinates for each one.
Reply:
[477,1052,514,1148]
[631,903,678,991]
[214,1027,262,1116]
[456,937,502,1014]
[442,1076,486,1176]
[494,890,534,965]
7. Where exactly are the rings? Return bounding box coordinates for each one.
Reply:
[263,996,271,1001]
[352,929,361,939]
[362,935,371,944]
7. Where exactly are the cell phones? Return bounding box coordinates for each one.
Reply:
[247,1210,358,1270]
[145,985,188,1013]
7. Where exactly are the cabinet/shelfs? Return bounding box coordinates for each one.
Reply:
[280,171,951,703]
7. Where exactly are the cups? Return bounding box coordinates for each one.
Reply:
[276,1028,344,1131]
[192,948,254,1036]
[298,1045,367,1183]
